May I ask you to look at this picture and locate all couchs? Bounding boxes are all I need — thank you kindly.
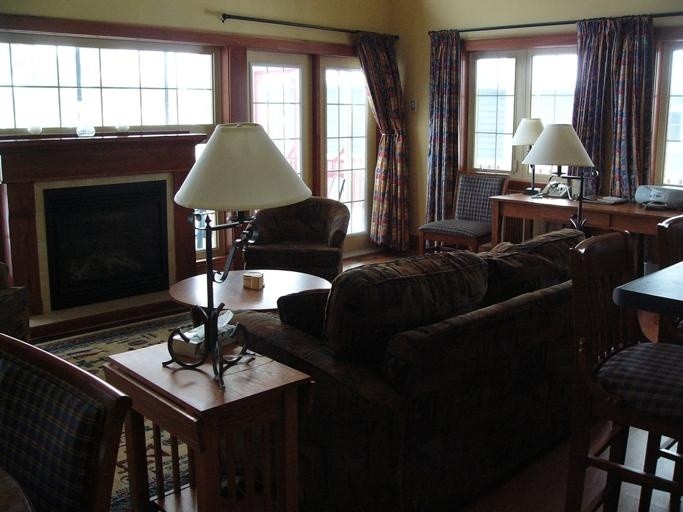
[227,227,585,512]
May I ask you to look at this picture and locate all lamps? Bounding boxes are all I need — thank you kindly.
[163,124,312,390]
[510,118,545,195]
[519,124,600,231]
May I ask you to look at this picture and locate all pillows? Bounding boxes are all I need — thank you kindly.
[323,249,489,346]
[475,227,585,306]
[277,289,330,331]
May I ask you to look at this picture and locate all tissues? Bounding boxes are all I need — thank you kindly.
[169,310,236,359]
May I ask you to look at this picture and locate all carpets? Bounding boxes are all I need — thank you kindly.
[30,310,195,511]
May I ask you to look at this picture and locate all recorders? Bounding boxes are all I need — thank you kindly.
[635,185,683,210]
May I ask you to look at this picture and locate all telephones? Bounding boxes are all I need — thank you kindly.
[542,181,570,198]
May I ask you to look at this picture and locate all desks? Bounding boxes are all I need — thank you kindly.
[489,192,683,250]
[611,260,683,512]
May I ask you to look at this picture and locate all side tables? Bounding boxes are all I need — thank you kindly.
[103,340,313,512]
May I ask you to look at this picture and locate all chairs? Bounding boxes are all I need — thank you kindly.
[0,332,132,512]
[418,171,510,258]
[638,215,683,268]
[565,230,683,512]
[241,196,350,282]
